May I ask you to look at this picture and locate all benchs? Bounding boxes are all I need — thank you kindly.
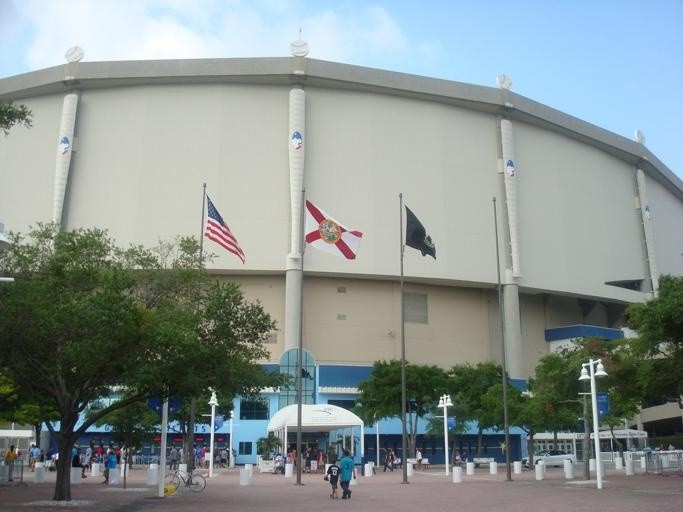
[406,458,431,471]
[472,456,494,466]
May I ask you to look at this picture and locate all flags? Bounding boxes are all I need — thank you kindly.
[204,194,245,263]
[304,199,363,262]
[404,203,436,260]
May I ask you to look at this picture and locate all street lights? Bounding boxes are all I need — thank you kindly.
[577,358,609,490]
[437,393,455,476]
[206,386,220,479]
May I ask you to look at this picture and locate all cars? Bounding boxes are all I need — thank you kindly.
[521,448,576,470]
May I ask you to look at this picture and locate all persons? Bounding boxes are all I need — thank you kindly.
[73,440,123,483]
[416,446,423,471]
[324,459,341,499]
[272,446,325,474]
[170,443,228,470]
[383,447,397,472]
[28,444,43,471]
[339,450,357,499]
[5,446,18,481]
[45,447,53,472]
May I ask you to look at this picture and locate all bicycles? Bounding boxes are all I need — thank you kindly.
[163,460,206,496]
